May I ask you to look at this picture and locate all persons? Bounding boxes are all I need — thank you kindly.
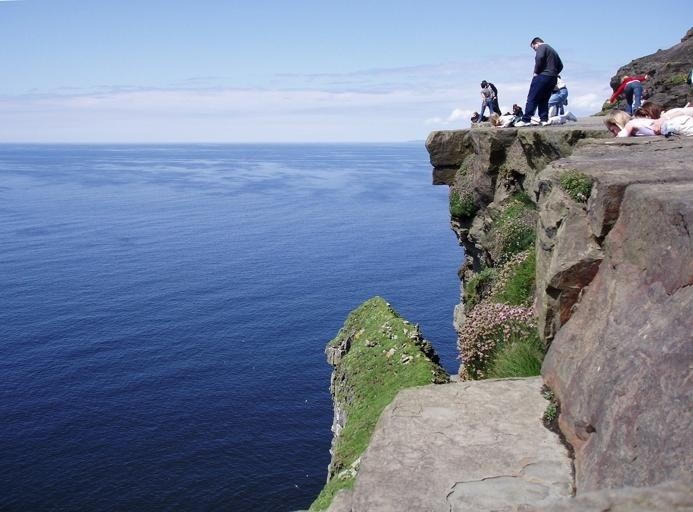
[602,75,693,138]
[471,75,577,128]
[514,37,563,127]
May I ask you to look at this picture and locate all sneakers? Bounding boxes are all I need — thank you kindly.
[567,111,577,122]
[514,121,531,128]
